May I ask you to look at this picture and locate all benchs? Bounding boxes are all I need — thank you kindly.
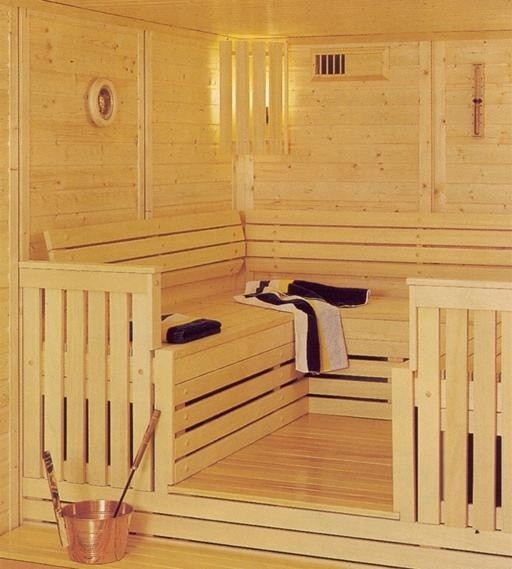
[242,210,512,419]
[44,210,309,483]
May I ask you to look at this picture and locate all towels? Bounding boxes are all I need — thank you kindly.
[130,313,222,344]
[233,293,349,375]
[245,280,369,308]
